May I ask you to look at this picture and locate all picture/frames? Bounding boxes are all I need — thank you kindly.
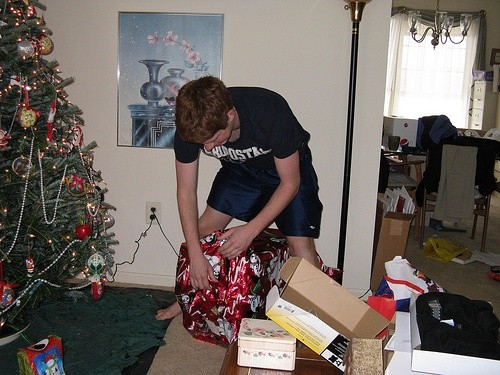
[117,11,224,149]
[490,48,500,65]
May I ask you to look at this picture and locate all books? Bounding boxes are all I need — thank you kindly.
[382,184,415,215]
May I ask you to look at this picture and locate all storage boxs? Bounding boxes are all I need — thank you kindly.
[382,115,418,150]
[371,199,416,291]
[473,70,493,81]
[238,257,387,375]
[384,297,500,375]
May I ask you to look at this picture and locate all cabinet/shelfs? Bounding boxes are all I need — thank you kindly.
[471,80,498,130]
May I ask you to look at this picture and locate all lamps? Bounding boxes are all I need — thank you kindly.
[410,0,473,50]
[337,0,373,286]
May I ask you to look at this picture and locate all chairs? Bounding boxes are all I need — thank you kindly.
[422,136,500,252]
[380,149,425,243]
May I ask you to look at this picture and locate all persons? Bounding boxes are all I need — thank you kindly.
[156,76,323,323]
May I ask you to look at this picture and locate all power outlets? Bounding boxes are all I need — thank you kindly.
[146,202,162,224]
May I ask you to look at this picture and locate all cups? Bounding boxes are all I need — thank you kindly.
[388,136,400,150]
[383,135,389,149]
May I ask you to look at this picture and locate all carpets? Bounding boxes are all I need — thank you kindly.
[0,283,177,375]
